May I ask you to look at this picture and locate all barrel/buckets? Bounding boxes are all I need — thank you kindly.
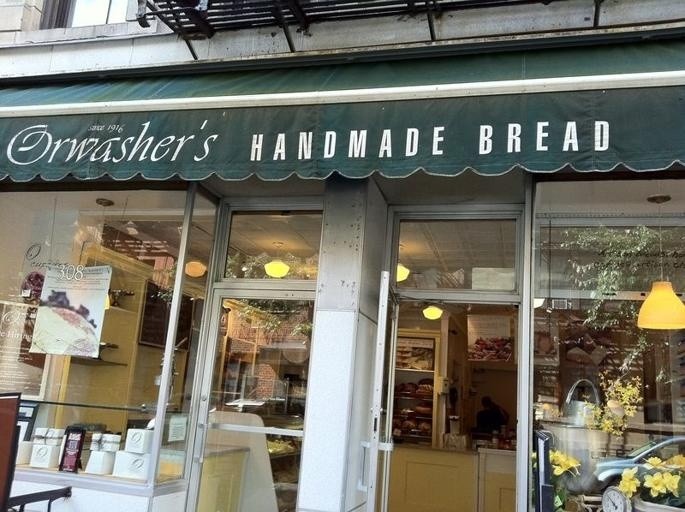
[540,424,609,495]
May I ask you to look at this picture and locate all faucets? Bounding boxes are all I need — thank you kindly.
[565,376,601,408]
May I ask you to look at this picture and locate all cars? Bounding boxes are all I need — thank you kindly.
[209,398,304,440]
[591,433,685,496]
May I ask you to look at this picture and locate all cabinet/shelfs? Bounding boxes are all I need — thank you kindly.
[48,252,279,452]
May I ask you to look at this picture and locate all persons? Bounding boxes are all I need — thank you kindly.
[477,395,506,437]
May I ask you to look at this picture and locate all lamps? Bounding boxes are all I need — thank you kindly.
[637,194,685,330]
